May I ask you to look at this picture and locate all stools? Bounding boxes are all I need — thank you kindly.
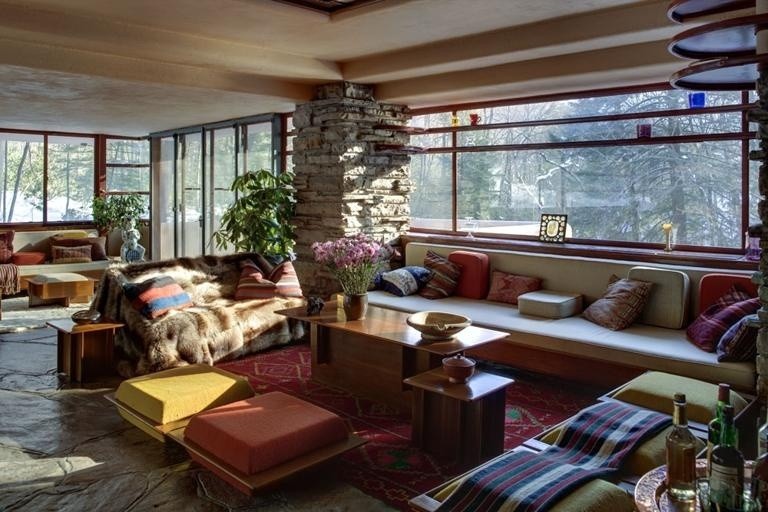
[24,273,95,308]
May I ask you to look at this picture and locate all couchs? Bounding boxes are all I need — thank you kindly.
[365,238,766,395]
[0,229,114,296]
[90,254,308,381]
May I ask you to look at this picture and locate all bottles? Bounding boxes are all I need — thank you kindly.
[749,453,768,511]
[706,383,740,484]
[758,423,768,457]
[666,393,697,502]
[710,406,744,512]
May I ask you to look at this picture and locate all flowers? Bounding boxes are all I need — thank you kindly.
[311,236,400,295]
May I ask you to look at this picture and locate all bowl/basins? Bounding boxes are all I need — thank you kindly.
[442,354,476,383]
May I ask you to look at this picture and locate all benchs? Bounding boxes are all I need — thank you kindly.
[103,364,371,499]
[403,370,749,512]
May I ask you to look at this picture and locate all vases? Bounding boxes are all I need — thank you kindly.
[343,293,370,324]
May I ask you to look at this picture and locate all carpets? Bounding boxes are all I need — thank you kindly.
[0,294,100,334]
[209,340,597,512]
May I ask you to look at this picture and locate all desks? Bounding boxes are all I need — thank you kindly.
[45,311,125,385]
[402,363,515,467]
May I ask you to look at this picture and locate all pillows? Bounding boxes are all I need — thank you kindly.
[0,229,110,264]
[123,274,193,319]
[233,255,305,299]
